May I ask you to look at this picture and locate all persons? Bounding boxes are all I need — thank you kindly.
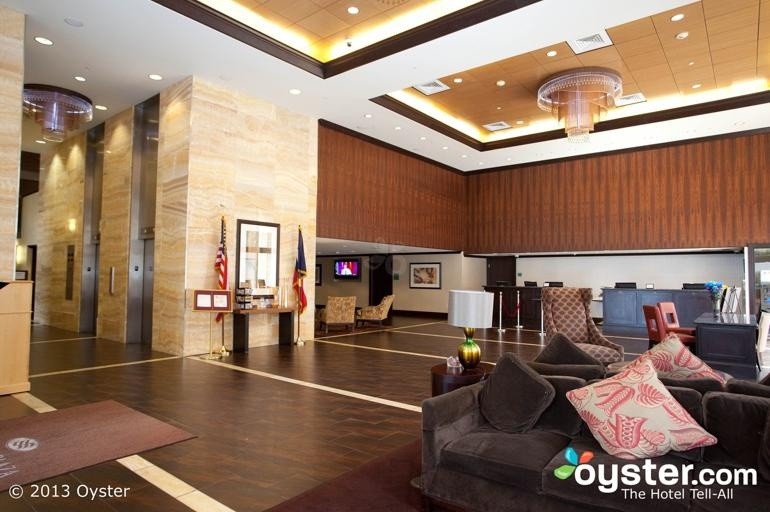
[340,261,353,276]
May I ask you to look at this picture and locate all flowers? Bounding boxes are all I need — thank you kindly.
[704,280,724,312]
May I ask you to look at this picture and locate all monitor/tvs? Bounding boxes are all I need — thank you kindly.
[334,258,360,280]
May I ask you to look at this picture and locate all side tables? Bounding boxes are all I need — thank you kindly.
[431,364,496,397]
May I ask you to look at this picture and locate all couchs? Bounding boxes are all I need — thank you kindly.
[420,360,770,512]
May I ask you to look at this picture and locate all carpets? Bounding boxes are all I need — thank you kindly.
[264,438,482,512]
[0,398,198,493]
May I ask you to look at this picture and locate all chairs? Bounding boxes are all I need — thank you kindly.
[524,281,537,287]
[545,282,563,287]
[541,287,624,366]
[496,281,510,287]
[614,283,636,289]
[682,283,706,290]
[643,302,697,357]
[356,295,397,329]
[317,296,357,334]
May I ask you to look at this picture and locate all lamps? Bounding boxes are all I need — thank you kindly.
[448,290,495,370]
[23,82,94,143]
[536,66,623,145]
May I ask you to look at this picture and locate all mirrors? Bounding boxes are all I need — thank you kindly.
[236,219,280,300]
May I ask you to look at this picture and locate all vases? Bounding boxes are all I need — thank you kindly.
[711,299,721,322]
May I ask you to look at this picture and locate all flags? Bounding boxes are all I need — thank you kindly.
[214,219,230,324]
[291,230,308,313]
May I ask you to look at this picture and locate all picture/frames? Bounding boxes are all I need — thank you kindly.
[409,262,441,289]
[315,263,322,287]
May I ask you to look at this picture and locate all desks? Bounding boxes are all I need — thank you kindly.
[693,312,759,381]
[232,308,297,353]
